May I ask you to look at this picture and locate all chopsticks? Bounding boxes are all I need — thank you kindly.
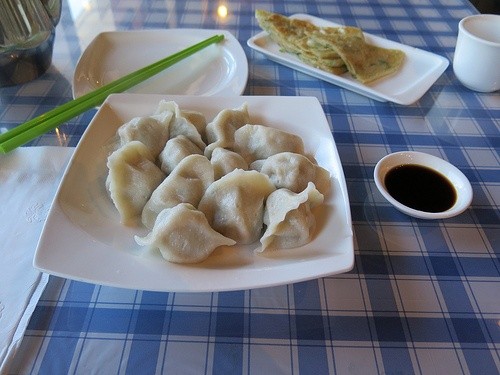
[0,34,227,153]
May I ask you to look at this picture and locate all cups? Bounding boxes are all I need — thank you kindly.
[452,15,500,92]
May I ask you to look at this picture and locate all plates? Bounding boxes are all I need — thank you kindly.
[72,28,249,112]
[35,94,354,292]
[372,151,475,218]
[246,13,449,105]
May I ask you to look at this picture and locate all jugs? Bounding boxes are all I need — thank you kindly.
[0,1,62,86]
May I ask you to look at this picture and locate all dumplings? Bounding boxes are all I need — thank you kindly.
[105,97,328,263]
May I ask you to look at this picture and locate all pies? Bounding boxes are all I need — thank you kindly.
[256,8,407,84]
